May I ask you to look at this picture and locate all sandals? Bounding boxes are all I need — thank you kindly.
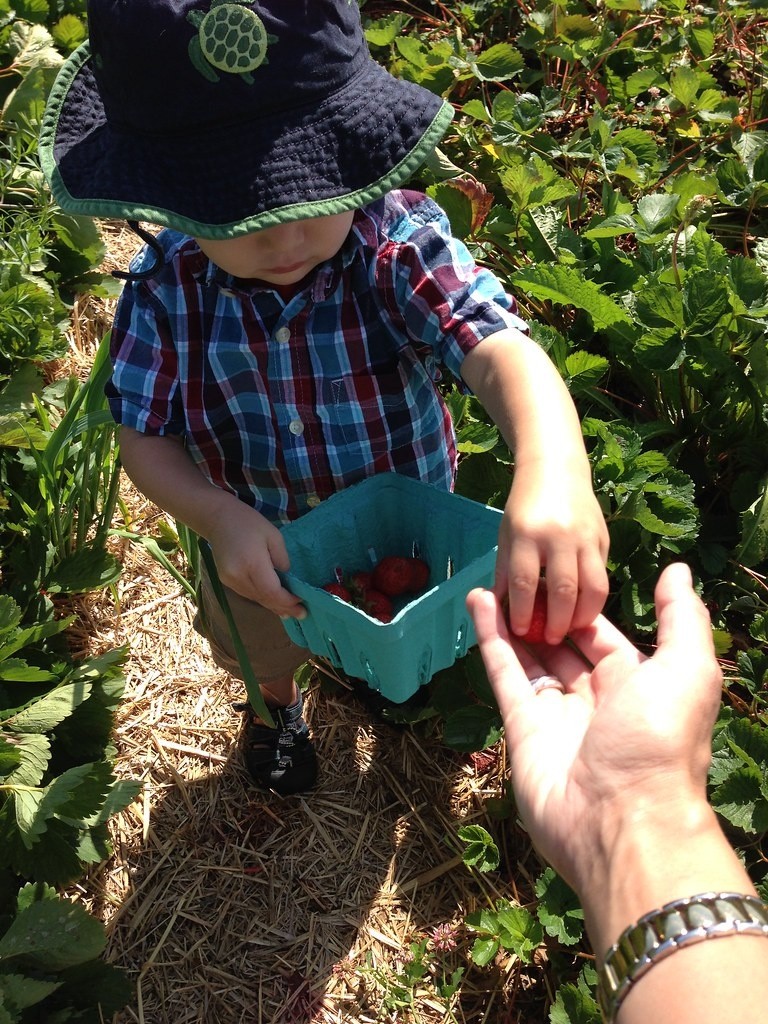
[347,678,432,727]
[232,683,317,795]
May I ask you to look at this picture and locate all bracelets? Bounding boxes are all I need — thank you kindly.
[595,890,768,1024]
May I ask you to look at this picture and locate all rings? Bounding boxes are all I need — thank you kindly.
[530,673,566,694]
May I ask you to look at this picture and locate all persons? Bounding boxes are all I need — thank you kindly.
[39,1,610,796]
[465,561,767,1024]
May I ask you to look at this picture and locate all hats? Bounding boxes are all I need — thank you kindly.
[39,0,456,241]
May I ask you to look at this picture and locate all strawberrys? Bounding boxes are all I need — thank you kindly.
[506,579,548,644]
[320,555,429,624]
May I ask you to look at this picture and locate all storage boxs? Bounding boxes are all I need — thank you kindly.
[273,473,507,703]
